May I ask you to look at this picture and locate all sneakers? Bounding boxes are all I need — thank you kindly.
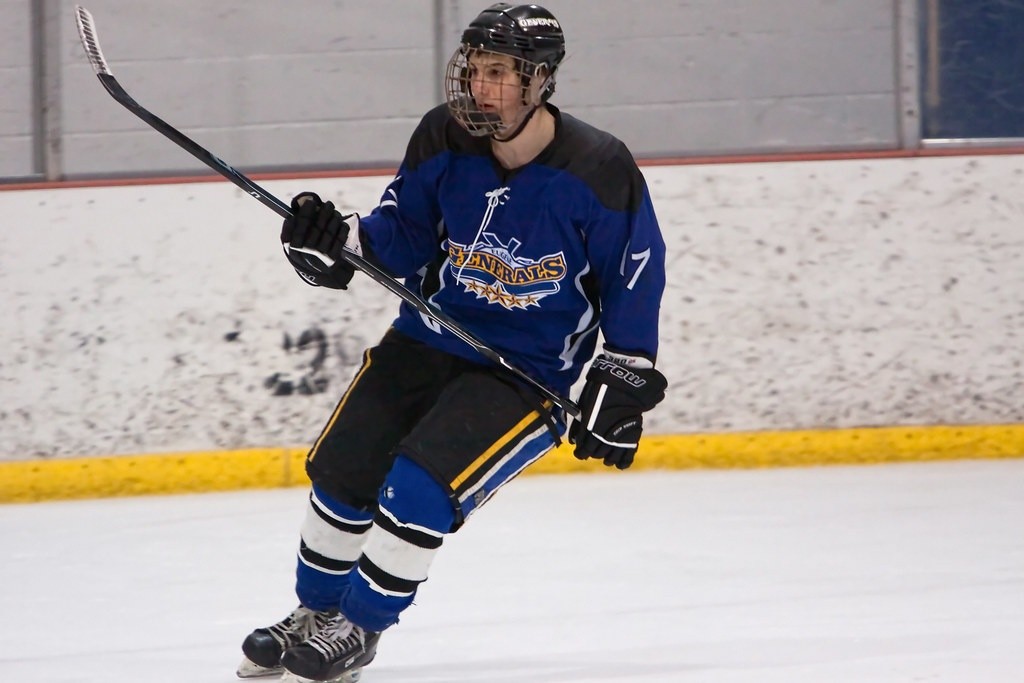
[236,606,338,677]
[279,612,381,683]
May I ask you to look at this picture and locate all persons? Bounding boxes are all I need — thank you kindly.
[236,0,667,683]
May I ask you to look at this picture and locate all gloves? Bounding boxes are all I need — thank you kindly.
[280,192,357,289]
[568,354,667,470]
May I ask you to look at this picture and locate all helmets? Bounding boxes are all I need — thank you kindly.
[445,3,566,137]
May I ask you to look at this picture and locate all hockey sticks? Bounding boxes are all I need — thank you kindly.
[71,3,584,422]
